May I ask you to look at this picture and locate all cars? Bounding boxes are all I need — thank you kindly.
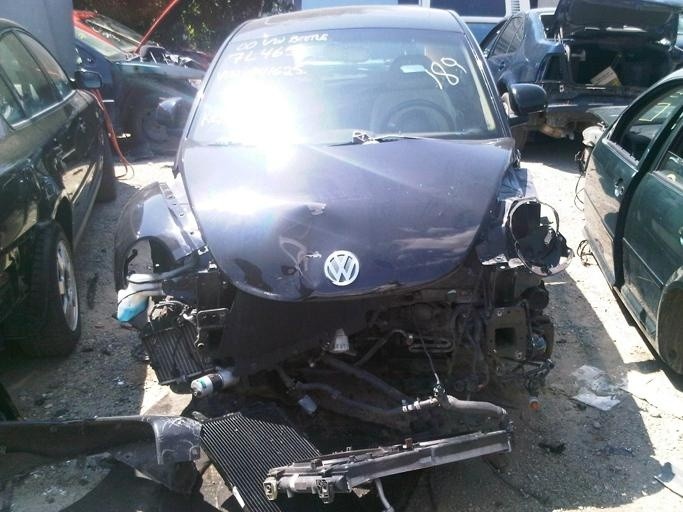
[574,67,683,378]
[479,0,682,156]
[73,0,214,156]
[460,16,505,44]
[113,5,581,430]
[1,18,117,360]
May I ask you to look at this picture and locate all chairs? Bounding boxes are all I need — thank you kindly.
[368,55,457,134]
